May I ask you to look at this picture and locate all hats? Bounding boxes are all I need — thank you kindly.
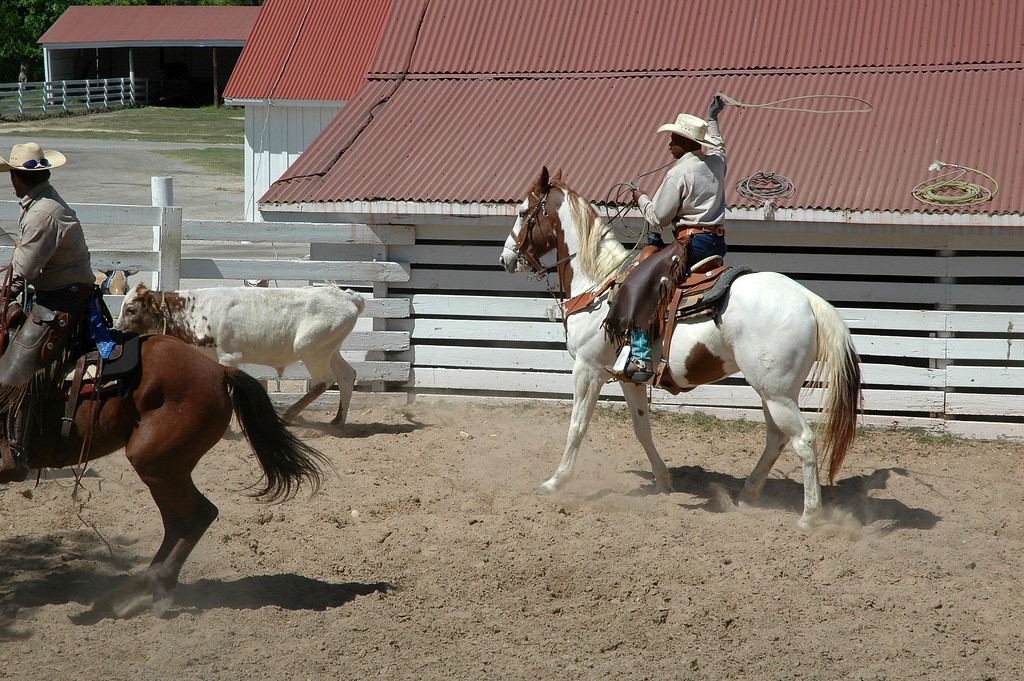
[655,111,717,150]
[0,143,68,173]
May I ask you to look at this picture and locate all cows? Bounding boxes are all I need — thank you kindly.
[113,282,366,439]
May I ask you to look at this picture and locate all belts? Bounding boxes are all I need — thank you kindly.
[671,225,727,240]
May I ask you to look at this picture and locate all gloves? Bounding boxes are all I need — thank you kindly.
[707,95,724,120]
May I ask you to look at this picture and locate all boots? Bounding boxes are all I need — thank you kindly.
[603,357,655,383]
[1,403,31,482]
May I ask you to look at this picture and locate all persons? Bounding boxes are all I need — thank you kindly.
[604,94,729,384]
[1,138,98,485]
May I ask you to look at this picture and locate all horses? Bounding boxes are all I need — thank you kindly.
[497,166,862,529]
[0,295,344,623]
